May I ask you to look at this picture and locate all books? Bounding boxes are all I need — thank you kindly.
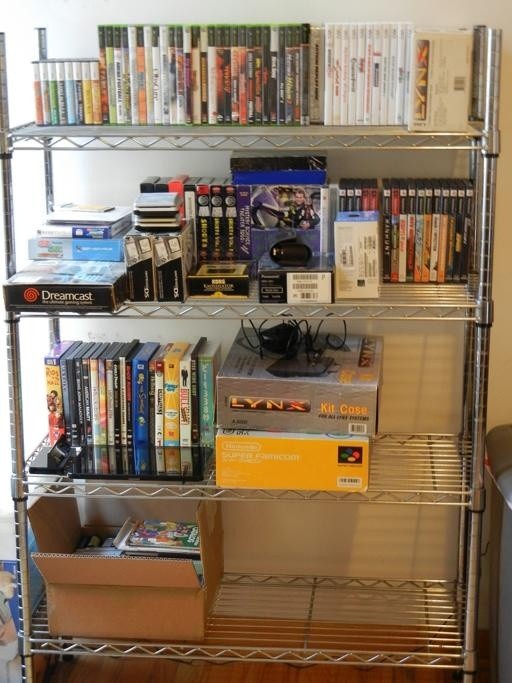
[214,324,385,434]
[230,156,327,280]
[216,429,368,492]
[44,342,220,480]
[29,205,132,262]
[321,178,474,283]
[217,328,383,435]
[215,430,371,493]
[25,474,223,644]
[74,522,202,576]
[31,24,474,132]
[126,175,235,301]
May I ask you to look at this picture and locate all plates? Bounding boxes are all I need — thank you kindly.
[0,23,503,683]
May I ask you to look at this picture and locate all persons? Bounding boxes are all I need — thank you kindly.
[278,191,320,229]
